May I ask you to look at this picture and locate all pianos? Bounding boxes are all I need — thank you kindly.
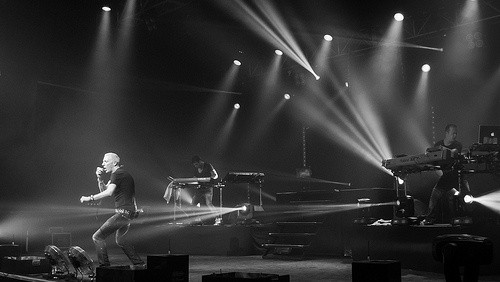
[223,171,265,184]
[382,143,458,173]
[167,176,213,189]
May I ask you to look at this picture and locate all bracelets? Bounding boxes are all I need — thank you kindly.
[91,195,94,201]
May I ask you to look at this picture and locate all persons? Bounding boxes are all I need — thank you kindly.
[420,125,470,225]
[191,156,222,226]
[80,152,146,278]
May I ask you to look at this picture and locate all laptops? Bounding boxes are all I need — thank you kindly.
[478,125,500,145]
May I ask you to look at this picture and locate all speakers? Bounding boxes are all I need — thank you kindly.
[352,260,402,282]
[95,254,189,282]
